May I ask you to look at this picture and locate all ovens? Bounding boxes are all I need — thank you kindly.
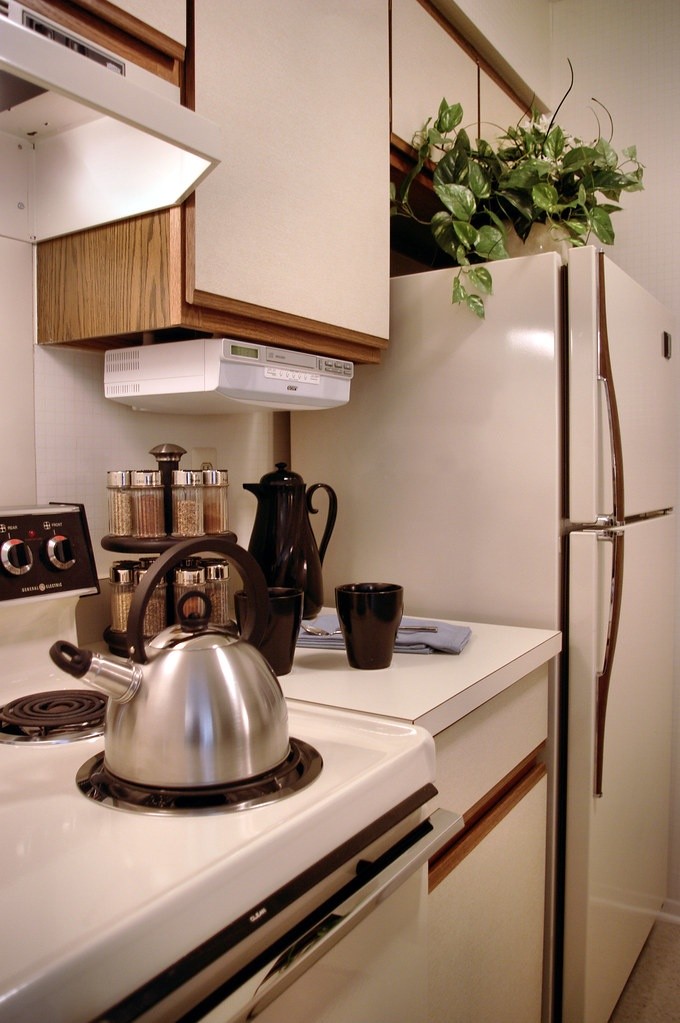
[128,784,464,1023]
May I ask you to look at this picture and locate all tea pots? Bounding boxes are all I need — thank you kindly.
[243,462,337,621]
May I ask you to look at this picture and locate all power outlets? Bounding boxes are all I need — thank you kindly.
[190,447,217,471]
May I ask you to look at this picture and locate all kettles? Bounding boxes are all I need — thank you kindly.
[49,537,291,788]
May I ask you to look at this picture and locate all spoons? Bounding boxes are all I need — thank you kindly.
[301,622,438,636]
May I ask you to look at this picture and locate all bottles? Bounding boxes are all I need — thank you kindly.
[171,470,230,537]
[174,556,230,627]
[108,470,164,537]
[110,558,165,637]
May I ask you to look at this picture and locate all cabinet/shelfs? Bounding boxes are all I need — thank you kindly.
[429,659,556,1023]
[0,0,397,364]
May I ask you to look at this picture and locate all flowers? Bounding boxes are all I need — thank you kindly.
[393,55,647,319]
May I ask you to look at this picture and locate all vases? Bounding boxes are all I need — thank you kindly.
[501,216,573,264]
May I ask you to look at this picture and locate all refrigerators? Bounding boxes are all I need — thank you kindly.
[285,244,680,1023]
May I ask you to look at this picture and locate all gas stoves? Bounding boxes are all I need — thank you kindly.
[0,502,438,1023]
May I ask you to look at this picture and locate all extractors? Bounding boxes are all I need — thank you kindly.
[0,0,226,244]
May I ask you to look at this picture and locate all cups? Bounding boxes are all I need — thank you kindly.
[233,588,304,676]
[335,582,403,671]
